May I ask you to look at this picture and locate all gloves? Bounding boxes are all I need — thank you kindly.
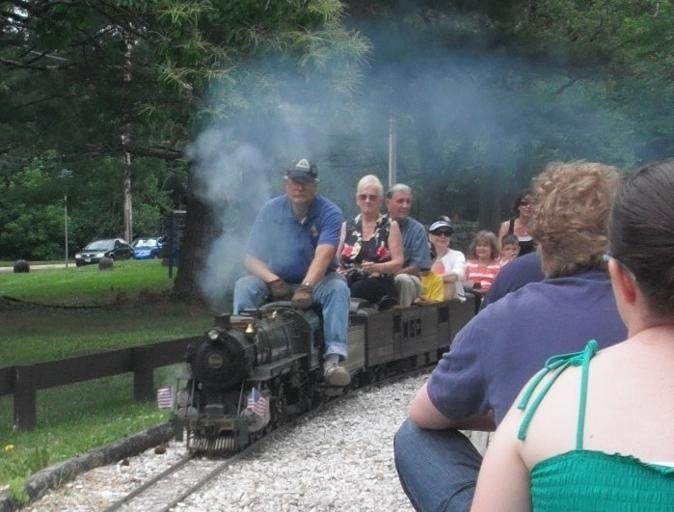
[291,284,314,311]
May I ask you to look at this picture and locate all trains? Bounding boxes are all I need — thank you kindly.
[169,286,484,460]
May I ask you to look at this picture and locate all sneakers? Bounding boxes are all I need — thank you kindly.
[323,364,351,398]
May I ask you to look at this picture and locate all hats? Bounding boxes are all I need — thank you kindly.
[427,215,454,232]
[285,156,318,183]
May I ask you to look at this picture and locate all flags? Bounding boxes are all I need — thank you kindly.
[247,388,265,416]
[157,388,171,410]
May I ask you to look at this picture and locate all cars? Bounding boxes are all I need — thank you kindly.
[74,235,168,267]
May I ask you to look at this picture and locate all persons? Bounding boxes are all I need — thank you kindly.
[469,158,674,512]
[233,158,351,387]
[394,160,630,512]
[336,175,544,312]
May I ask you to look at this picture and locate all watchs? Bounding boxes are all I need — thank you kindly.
[301,281,313,289]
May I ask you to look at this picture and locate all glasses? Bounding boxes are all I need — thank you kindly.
[431,229,453,236]
[519,201,533,206]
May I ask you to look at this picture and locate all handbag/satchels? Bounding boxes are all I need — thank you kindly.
[267,278,292,301]
